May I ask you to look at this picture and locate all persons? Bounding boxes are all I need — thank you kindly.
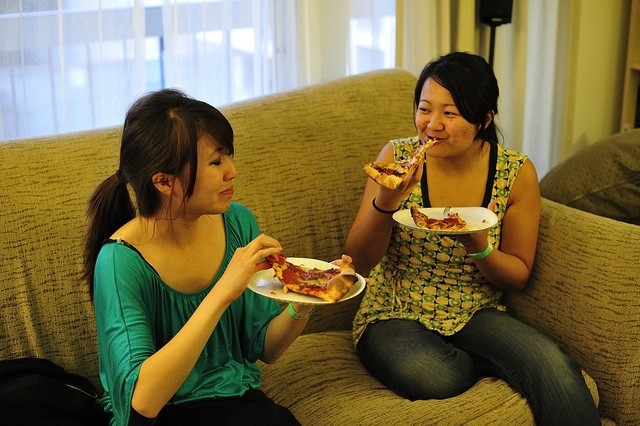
[80,89,354,426]
[345,51,601,426]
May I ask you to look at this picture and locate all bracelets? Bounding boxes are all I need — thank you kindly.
[286,302,317,321]
[372,197,402,215]
[467,244,493,260]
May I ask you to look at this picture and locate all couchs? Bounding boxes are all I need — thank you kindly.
[0,71,640,425]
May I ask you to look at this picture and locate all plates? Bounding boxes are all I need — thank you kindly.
[247,257,367,306]
[392,206,499,235]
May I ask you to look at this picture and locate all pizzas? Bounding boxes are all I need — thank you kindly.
[364,138,440,189]
[263,251,355,304]
[410,204,467,232]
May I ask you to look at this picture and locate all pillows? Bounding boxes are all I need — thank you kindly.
[540,126,640,226]
